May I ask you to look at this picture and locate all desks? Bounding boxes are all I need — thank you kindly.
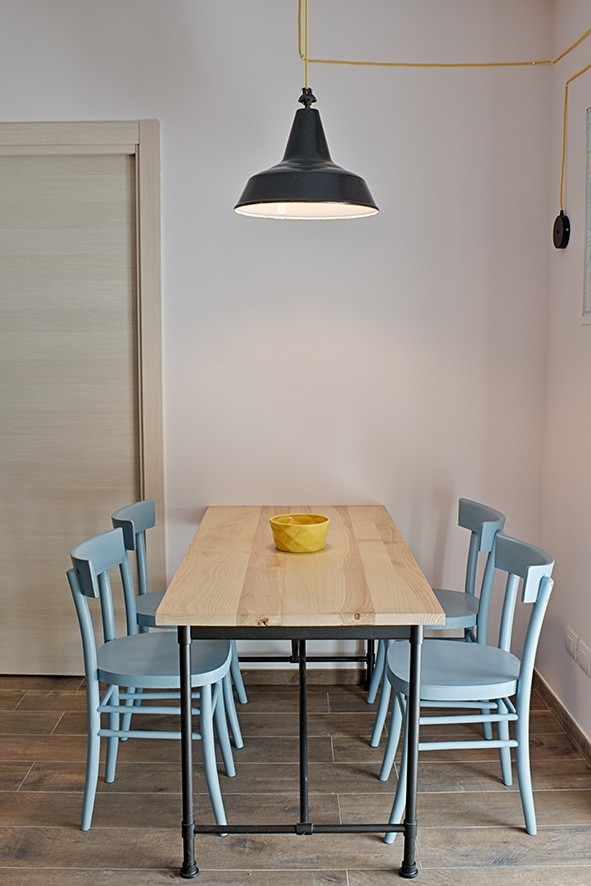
[155,504,446,881]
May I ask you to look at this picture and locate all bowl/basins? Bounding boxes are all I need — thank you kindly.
[269,513,330,553]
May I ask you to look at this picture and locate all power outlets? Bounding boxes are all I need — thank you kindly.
[564,626,591,677]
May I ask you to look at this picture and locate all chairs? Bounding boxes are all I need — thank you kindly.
[380,534,555,844]
[112,498,247,750]
[367,499,506,746]
[66,527,230,838]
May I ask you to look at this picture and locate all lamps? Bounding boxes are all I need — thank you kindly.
[233,0,380,220]
